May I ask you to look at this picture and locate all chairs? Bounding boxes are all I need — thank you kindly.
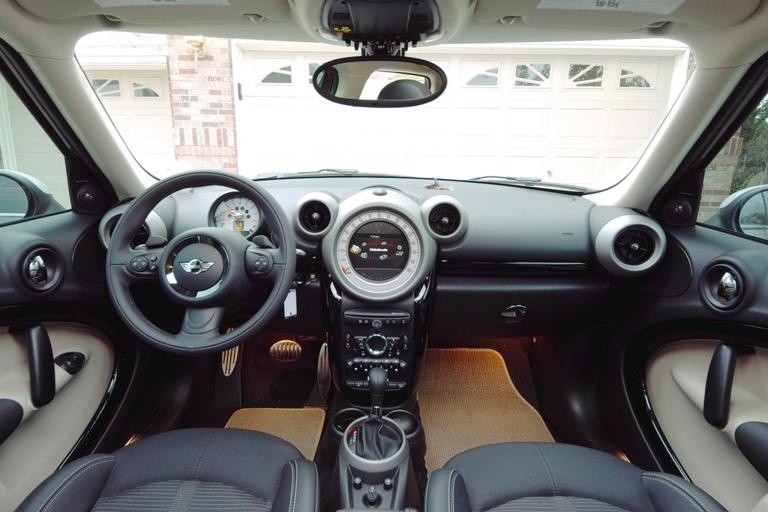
[422,440,729,511]
[14,427,321,512]
